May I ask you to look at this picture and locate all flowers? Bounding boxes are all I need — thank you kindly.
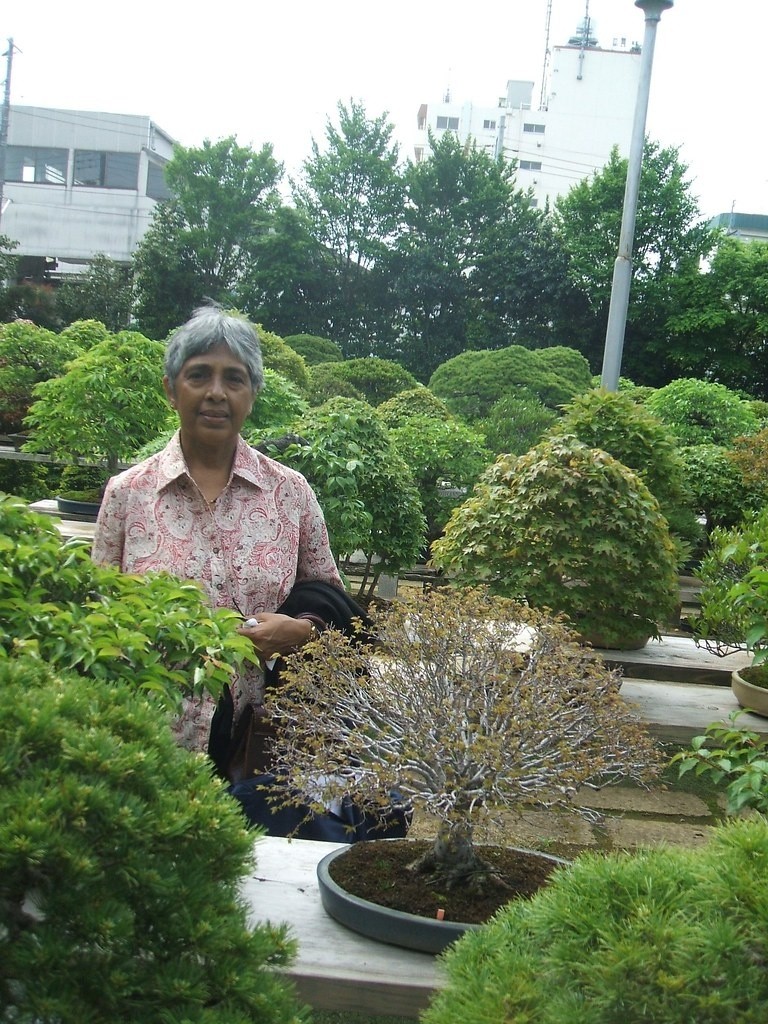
[253,585,671,898]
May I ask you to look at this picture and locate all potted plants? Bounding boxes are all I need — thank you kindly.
[21,342,172,515]
[688,504,768,716]
[426,435,679,649]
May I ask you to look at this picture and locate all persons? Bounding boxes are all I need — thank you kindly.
[91,307,343,794]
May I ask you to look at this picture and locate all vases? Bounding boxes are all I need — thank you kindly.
[318,838,574,952]
